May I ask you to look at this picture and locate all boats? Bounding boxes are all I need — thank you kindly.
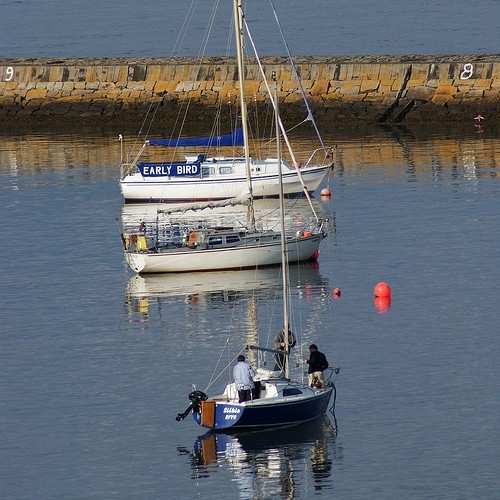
[177,418,336,500]
[169,68,345,434]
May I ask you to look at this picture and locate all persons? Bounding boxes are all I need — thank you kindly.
[304,344,329,389]
[274,323,296,372]
[232,355,255,404]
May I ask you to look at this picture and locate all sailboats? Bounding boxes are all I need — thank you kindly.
[125,261,329,369]
[122,198,329,237]
[119,1,338,201]
[116,1,332,277]
